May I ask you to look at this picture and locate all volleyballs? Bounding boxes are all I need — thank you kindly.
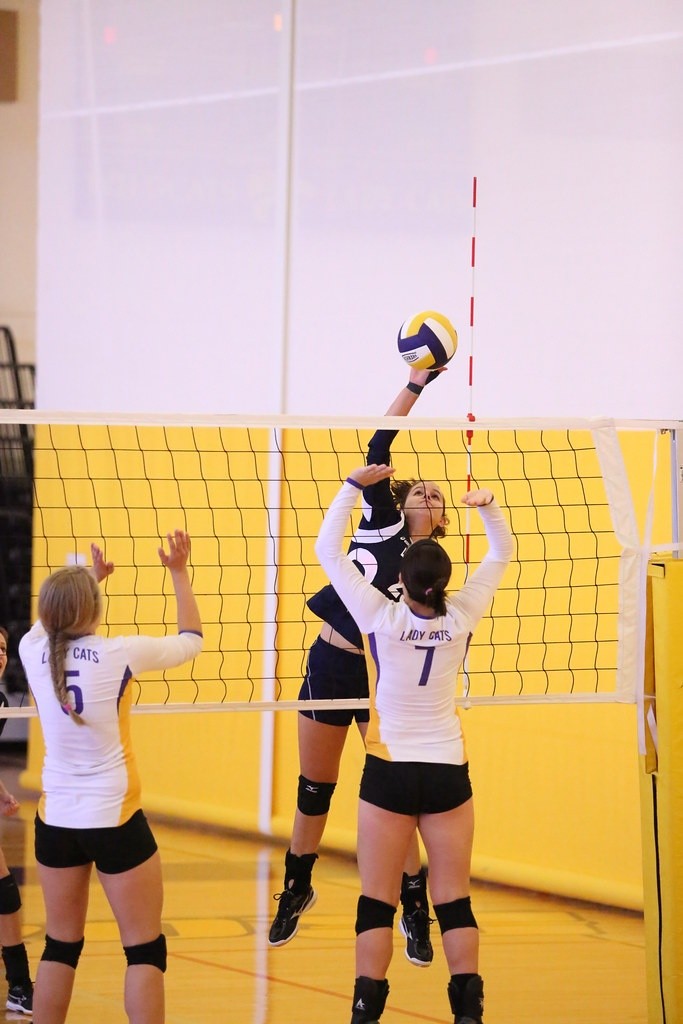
[396,309,459,372]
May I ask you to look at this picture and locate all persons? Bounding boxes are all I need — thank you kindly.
[267,363,451,967]
[0,627,36,1016]
[18,529,205,1024]
[314,463,512,1024]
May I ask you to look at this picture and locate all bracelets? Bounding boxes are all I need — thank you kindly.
[405,381,424,395]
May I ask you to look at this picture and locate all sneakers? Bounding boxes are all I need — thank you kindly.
[268,883,319,947]
[398,900,434,967]
[5,987,33,1014]
